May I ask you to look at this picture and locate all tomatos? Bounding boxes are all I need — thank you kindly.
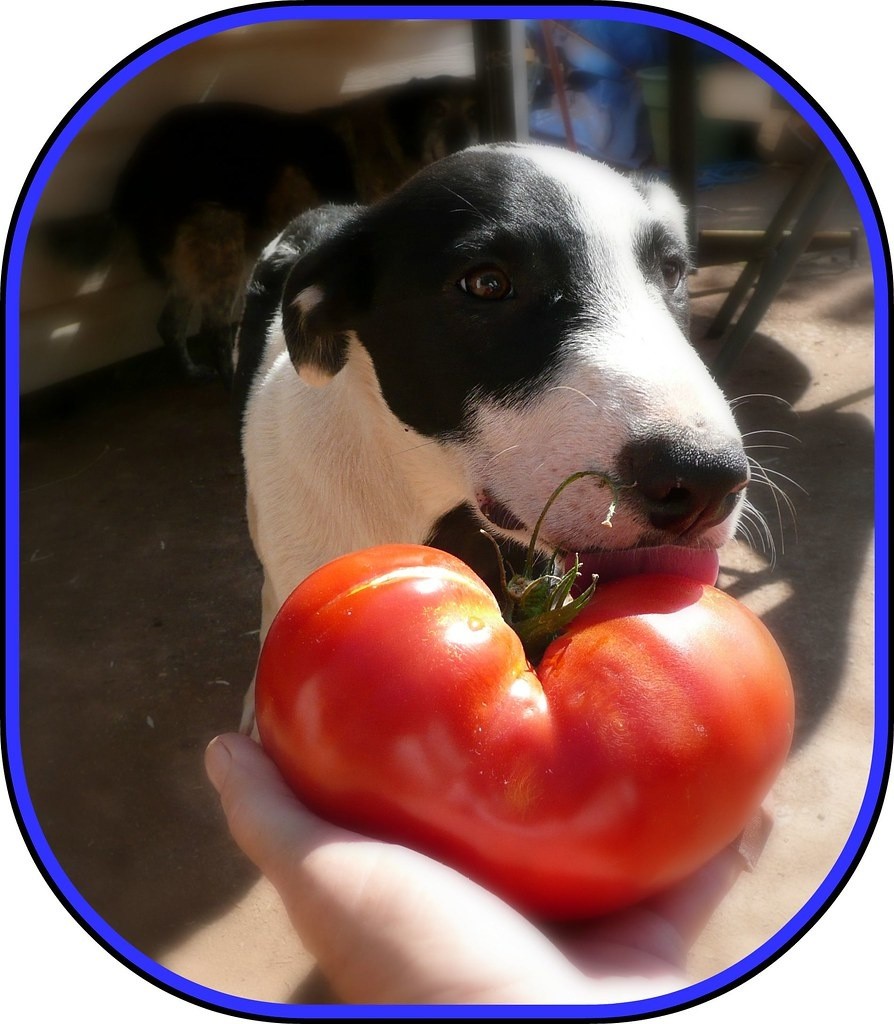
[253,465,793,927]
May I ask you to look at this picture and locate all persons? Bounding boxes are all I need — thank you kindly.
[205,735,773,1004]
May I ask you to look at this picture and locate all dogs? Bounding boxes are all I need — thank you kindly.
[30,68,749,760]
